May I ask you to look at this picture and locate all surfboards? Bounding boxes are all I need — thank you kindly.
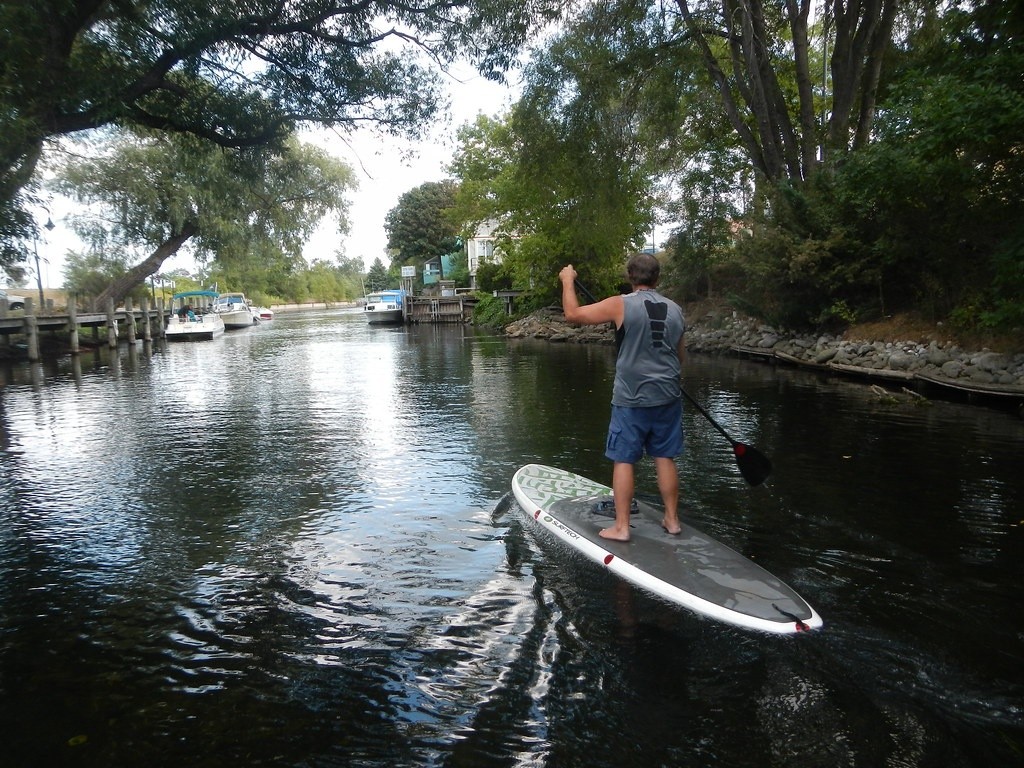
[510,463,824,635]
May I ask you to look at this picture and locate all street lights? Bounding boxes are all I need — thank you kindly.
[27,205,56,312]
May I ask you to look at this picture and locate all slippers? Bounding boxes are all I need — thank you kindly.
[630,498,639,514]
[591,499,616,517]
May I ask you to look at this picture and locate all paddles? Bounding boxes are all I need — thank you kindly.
[572,278,774,488]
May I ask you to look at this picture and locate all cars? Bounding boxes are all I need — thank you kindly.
[0,290,35,313]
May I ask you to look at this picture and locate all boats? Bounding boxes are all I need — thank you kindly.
[362,277,407,326]
[212,281,256,328]
[162,290,228,342]
[250,306,280,322]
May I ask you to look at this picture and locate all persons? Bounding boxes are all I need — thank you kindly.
[558,252,683,540]
[178,303,213,322]
